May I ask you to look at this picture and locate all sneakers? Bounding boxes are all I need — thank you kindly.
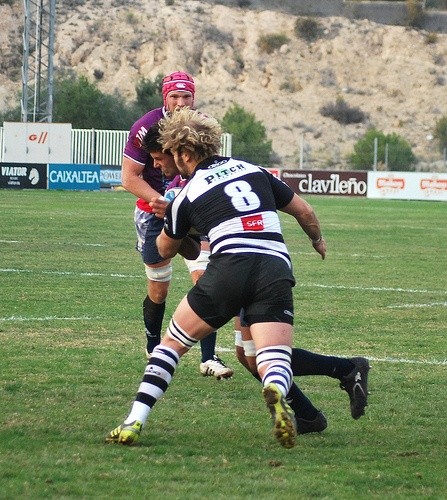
[339,357,372,420]
[262,383,297,449]
[146,348,153,364]
[199,355,233,380]
[293,409,327,434]
[105,420,143,444]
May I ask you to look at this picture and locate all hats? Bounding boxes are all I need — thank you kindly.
[162,72,194,110]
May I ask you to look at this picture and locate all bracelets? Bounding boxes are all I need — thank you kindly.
[309,235,323,243]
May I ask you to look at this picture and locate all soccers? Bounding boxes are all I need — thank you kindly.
[163,187,183,203]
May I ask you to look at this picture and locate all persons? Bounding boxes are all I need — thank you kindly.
[102,104,328,447]
[142,127,372,434]
[121,71,236,383]
[377,160,392,171]
